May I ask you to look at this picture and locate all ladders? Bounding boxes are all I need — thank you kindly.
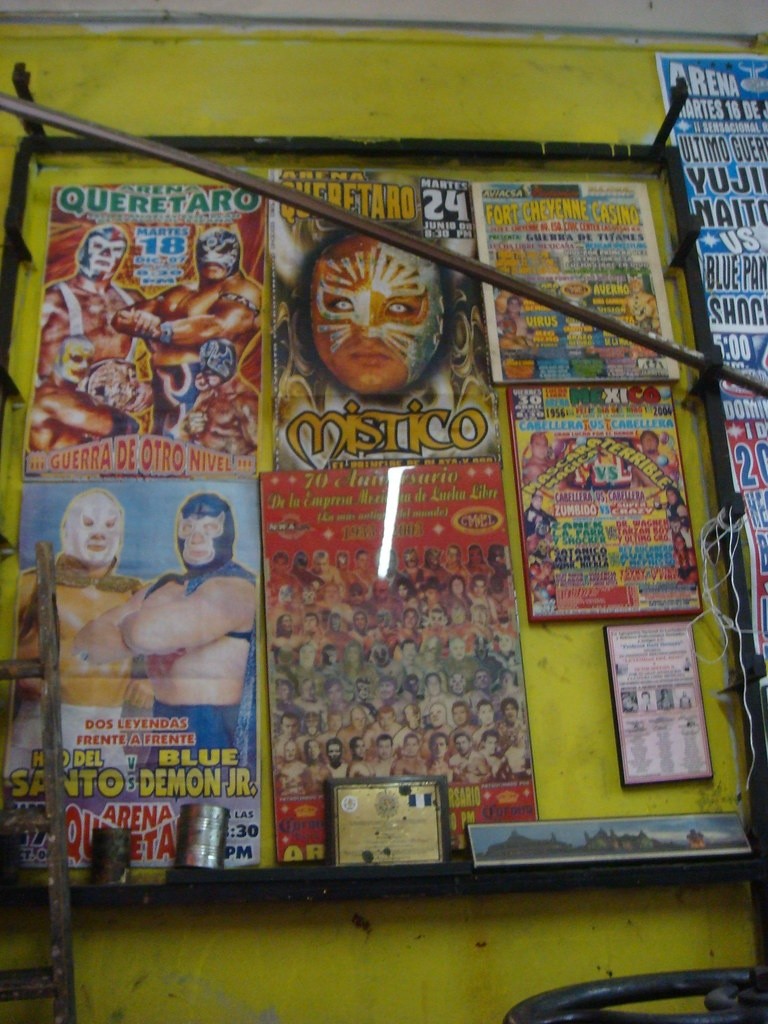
[1,540,80,1024]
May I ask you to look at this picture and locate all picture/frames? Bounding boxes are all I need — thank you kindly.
[464,810,754,873]
[323,774,452,866]
[603,622,715,790]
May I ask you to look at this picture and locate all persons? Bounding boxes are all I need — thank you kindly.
[14,226,531,792]
[634,431,697,582]
[622,688,692,712]
[523,432,557,600]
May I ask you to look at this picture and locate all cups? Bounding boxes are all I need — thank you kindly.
[175,804,229,871]
